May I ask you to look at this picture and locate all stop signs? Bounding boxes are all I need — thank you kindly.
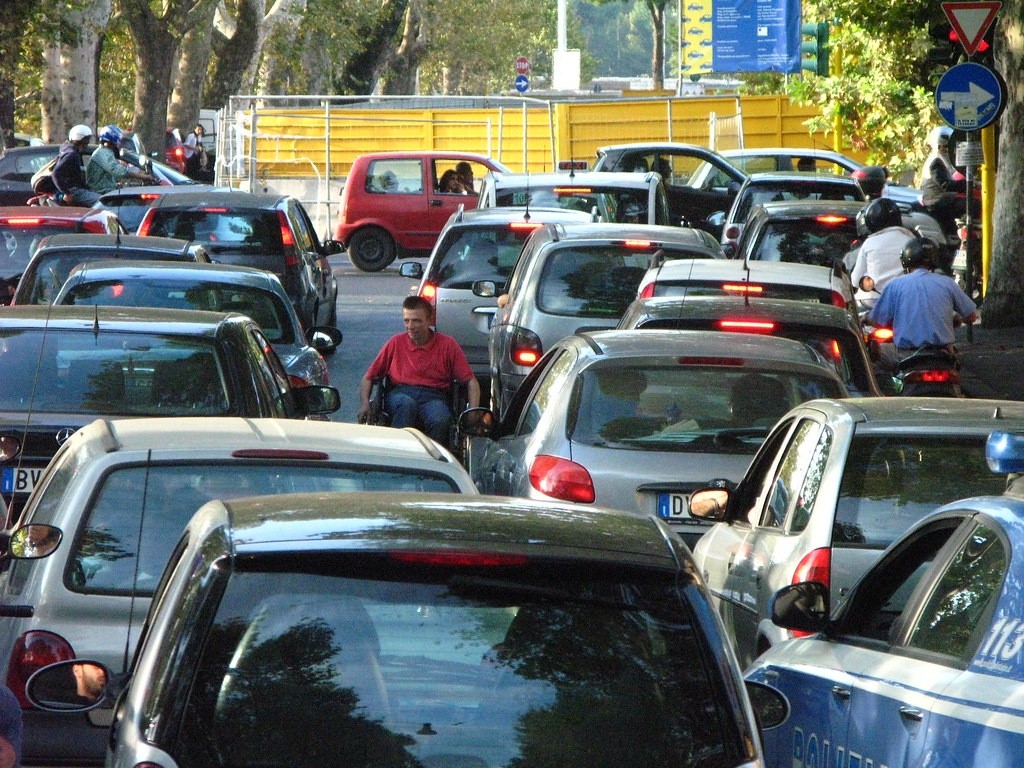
[517,56,530,75]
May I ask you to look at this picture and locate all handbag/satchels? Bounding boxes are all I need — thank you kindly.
[31,153,60,196]
[200,150,207,166]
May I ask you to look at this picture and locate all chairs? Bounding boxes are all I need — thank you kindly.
[217,603,389,768]
[68,359,124,402]
[0,344,59,402]
[862,448,909,529]
[175,213,209,241]
[777,233,815,262]
[591,372,647,430]
[91,477,189,585]
[149,353,216,409]
[481,600,669,768]
[728,374,791,430]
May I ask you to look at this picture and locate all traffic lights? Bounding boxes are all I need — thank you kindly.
[799,21,830,79]
[975,19,1000,64]
[930,20,959,67]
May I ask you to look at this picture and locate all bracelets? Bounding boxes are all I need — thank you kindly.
[711,497,719,516]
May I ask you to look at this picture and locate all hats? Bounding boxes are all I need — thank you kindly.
[99,125,125,146]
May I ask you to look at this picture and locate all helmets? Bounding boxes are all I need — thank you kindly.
[865,198,902,233]
[928,126,954,154]
[69,125,92,142]
[852,165,886,199]
[901,237,939,269]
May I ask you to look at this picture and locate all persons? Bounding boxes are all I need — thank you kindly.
[27,525,61,558]
[858,238,976,360]
[73,664,106,706]
[52,125,100,208]
[850,199,917,318]
[693,496,728,518]
[86,125,154,194]
[860,276,873,291]
[464,239,498,275]
[798,158,816,172]
[920,126,967,277]
[359,296,480,447]
[651,158,711,190]
[440,162,477,194]
[380,171,410,193]
[0,437,19,462]
[184,124,203,180]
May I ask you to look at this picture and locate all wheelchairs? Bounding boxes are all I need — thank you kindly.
[361,374,467,470]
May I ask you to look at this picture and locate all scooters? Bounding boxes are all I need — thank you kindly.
[857,271,980,398]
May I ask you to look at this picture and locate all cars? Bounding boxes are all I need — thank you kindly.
[747,422,1024,767]
[686,396,1024,681]
[24,488,792,768]
[1,95,982,559]
[0,415,513,768]
